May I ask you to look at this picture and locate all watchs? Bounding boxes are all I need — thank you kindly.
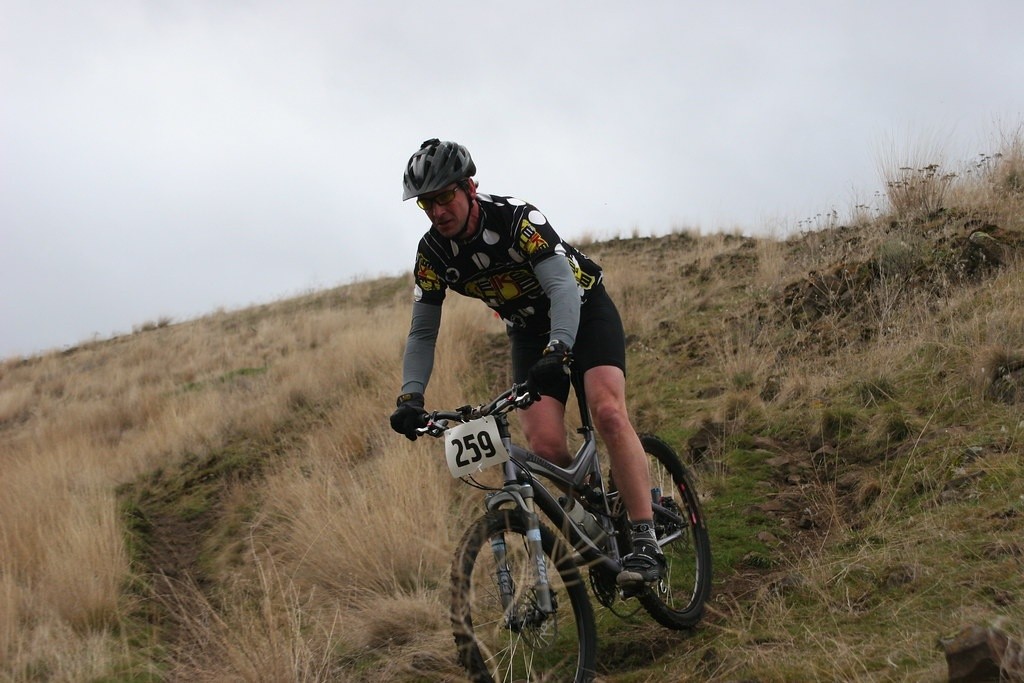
[547,340,563,347]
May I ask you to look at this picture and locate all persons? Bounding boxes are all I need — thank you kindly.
[389,138,669,583]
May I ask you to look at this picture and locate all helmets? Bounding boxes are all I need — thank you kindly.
[402,138,474,202]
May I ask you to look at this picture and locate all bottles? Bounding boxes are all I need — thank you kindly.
[557,496,614,555]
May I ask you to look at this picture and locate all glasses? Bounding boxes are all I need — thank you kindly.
[417,179,468,211]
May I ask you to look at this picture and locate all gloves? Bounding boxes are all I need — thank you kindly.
[391,393,430,441]
[528,348,566,402]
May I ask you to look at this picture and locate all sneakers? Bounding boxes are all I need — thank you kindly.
[616,536,667,586]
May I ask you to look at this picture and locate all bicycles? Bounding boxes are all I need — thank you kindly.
[413,358,712,683]
